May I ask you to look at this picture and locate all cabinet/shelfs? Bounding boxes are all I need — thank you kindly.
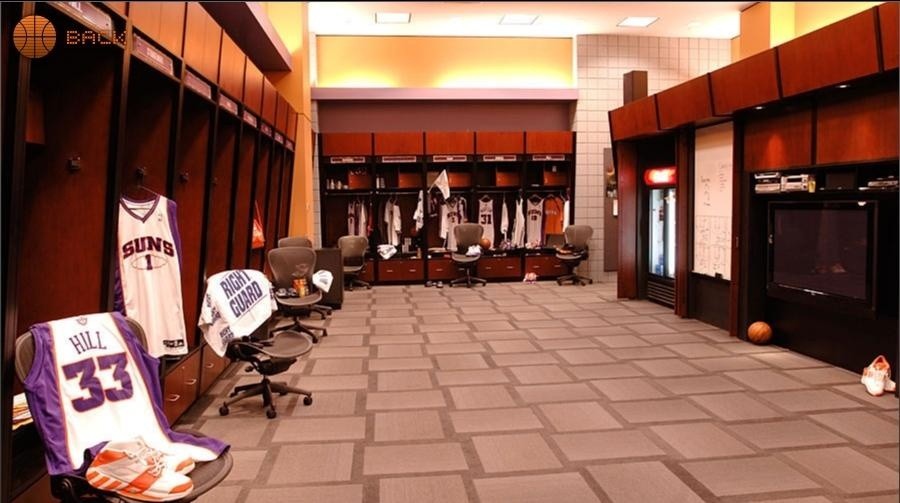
[317,129,578,283]
[1,0,298,501]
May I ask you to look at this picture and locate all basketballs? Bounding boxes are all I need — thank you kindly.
[480,239,490,248]
[13,15,56,59]
[747,322,771,344]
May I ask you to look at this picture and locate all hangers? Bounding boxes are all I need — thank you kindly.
[347,184,574,208]
[119,166,157,211]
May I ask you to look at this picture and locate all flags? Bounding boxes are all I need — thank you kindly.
[434,170,451,201]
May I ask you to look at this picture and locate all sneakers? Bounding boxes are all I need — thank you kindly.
[860,356,895,397]
[425,280,443,288]
[276,285,298,297]
[558,243,575,254]
[523,272,536,284]
[85,432,194,502]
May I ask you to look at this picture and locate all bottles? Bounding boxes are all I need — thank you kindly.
[417,248,421,258]
[809,176,816,193]
[292,276,309,298]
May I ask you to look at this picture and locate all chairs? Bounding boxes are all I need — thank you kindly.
[13,233,323,503]
[337,234,373,291]
[554,223,596,287]
[450,223,488,288]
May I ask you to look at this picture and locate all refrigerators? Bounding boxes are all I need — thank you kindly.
[643,163,676,310]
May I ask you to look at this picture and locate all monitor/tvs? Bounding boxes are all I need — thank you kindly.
[765,199,880,322]
